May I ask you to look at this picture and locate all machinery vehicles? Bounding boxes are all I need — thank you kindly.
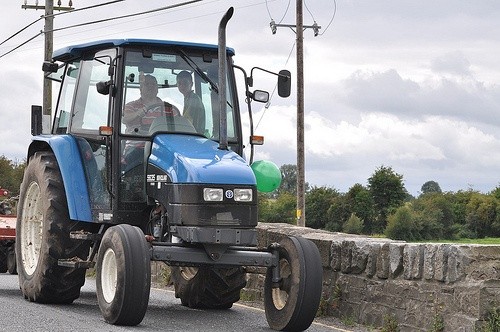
[0,185,21,274]
[13,6,324,332]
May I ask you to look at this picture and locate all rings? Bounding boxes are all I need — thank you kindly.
[138,115,140,117]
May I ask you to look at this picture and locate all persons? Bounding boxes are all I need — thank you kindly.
[176,71,205,134]
[121,74,180,171]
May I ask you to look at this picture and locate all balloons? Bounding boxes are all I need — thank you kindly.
[251,160,282,192]
